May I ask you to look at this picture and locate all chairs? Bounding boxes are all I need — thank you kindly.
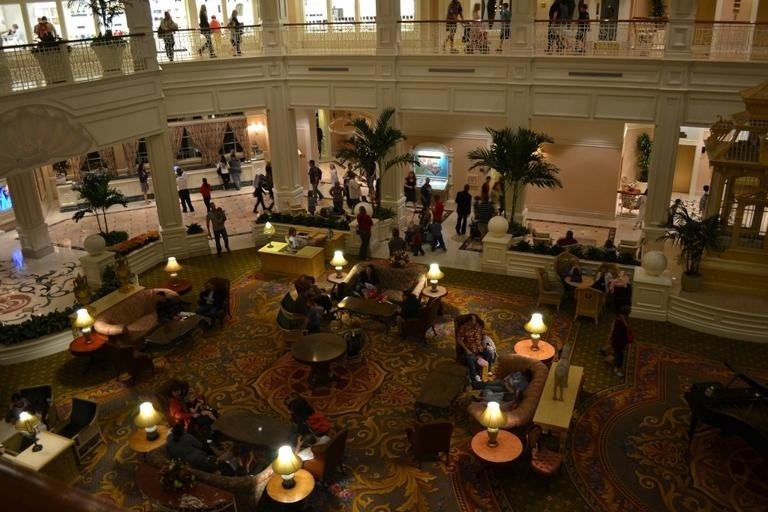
[406,420,452,465]
[398,298,441,338]
[21,383,58,432]
[534,230,640,323]
[51,397,106,465]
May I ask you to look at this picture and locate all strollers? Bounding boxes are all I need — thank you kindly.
[404,209,424,254]
[460,22,491,56]
[552,27,583,56]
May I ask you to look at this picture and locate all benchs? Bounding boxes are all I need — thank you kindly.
[156,378,211,438]
[291,423,348,484]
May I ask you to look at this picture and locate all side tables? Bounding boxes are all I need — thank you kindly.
[69,333,113,377]
[267,468,316,512]
[156,279,193,297]
[131,426,172,472]
[516,338,556,363]
[470,429,523,481]
[327,272,349,297]
[421,285,448,314]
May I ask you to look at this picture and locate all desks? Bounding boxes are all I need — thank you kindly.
[417,360,470,417]
[144,311,203,350]
[257,230,346,281]
[533,360,584,452]
[214,410,302,450]
[327,296,401,327]
[21,428,82,483]
[292,333,348,386]
[69,285,145,337]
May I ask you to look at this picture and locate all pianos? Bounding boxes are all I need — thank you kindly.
[684,361,766,449]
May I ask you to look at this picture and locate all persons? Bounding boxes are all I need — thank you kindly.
[226,10,244,56]
[556,230,577,251]
[698,184,710,219]
[632,188,648,231]
[196,5,217,59]
[176,170,195,213]
[155,291,181,324]
[276,263,421,340]
[209,15,222,50]
[195,280,226,327]
[456,314,494,381]
[7,16,58,47]
[167,384,333,474]
[316,123,324,153]
[671,198,681,218]
[591,263,633,312]
[137,163,151,205]
[597,305,632,378]
[441,0,591,55]
[160,11,177,62]
[604,239,614,248]
[5,392,33,423]
[562,255,587,310]
[474,368,534,393]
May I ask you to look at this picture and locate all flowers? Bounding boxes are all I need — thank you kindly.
[103,229,162,258]
[20,273,91,345]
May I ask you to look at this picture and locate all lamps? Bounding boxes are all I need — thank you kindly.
[425,263,444,292]
[274,445,303,490]
[330,250,346,278]
[165,256,183,285]
[135,402,163,441]
[478,401,506,447]
[75,308,96,343]
[524,314,548,350]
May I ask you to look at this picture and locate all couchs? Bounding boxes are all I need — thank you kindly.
[467,353,550,434]
[93,289,182,350]
[196,276,231,328]
[166,440,280,482]
[339,261,429,305]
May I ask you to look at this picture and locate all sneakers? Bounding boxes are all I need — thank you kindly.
[254,208,272,212]
[222,185,240,190]
[314,195,323,203]
[458,231,467,235]
[182,209,194,212]
[413,246,447,256]
[601,344,626,378]
[218,249,231,257]
